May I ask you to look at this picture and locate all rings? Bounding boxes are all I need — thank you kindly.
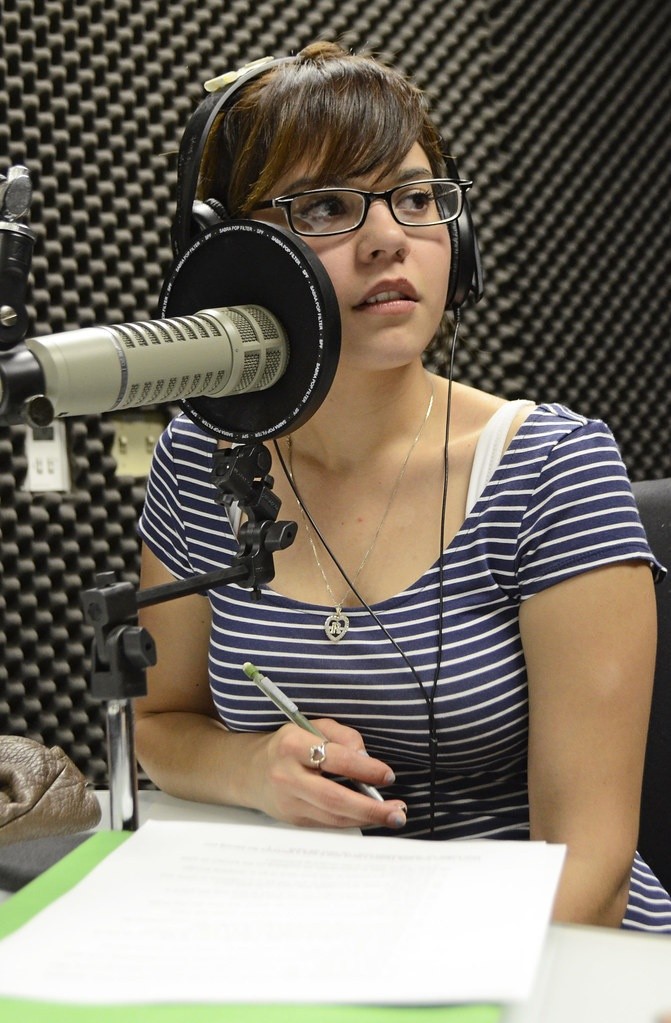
[310,740,331,772]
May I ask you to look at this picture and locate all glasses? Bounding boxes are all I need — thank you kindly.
[255,178,474,238]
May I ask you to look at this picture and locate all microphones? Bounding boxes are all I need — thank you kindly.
[0,304,289,427]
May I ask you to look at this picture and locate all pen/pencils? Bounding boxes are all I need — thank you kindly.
[242,660,383,802]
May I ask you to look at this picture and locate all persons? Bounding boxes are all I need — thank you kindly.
[130,42,671,934]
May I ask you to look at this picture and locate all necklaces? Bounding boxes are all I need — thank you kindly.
[286,375,435,643]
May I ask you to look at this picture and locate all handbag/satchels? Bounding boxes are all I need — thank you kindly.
[0,736,103,842]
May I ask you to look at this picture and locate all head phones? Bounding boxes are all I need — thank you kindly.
[170,57,483,310]
